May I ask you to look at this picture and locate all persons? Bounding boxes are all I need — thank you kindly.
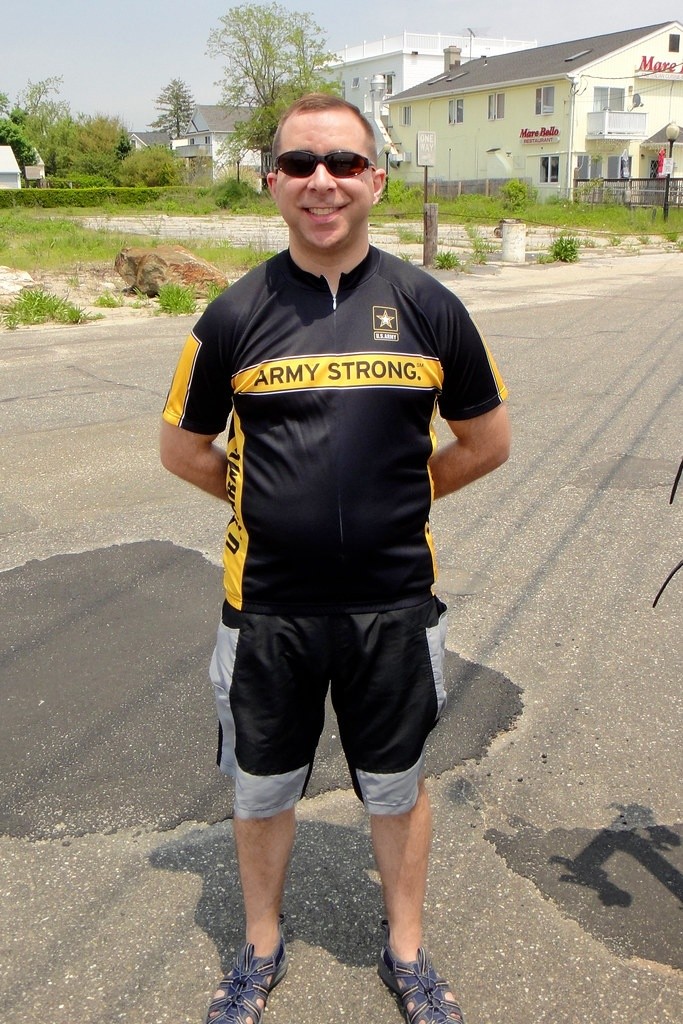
[160,94,512,1024]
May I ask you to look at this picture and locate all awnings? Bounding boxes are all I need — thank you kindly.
[641,123,683,146]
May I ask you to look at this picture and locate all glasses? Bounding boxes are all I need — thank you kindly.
[273,151,377,178]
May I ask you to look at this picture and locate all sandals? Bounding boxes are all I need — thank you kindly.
[377,919,465,1024]
[207,914,289,1024]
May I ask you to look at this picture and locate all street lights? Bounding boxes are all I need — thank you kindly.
[235,155,241,182]
[662,122,680,222]
[383,144,391,194]
